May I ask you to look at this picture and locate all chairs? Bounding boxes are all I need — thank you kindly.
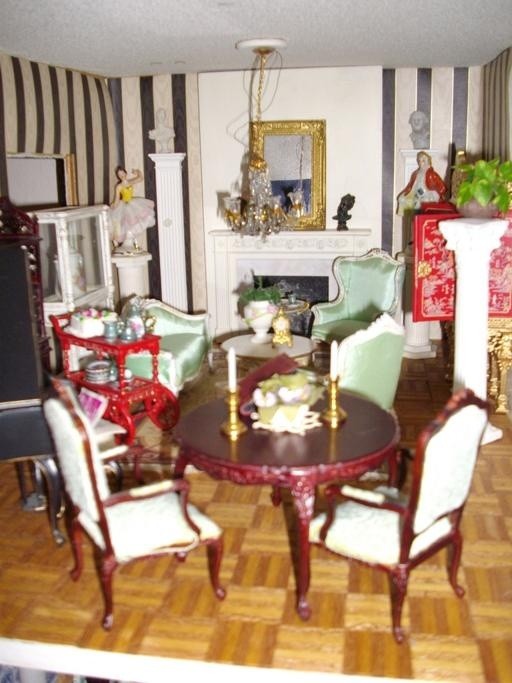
[305,387,490,644]
[39,375,226,630]
[310,247,407,411]
[118,296,218,401]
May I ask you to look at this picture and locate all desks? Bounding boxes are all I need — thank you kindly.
[172,382,402,620]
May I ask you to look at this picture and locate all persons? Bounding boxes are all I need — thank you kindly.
[408,109,431,151]
[394,149,445,213]
[111,164,155,251]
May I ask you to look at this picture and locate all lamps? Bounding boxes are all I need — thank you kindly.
[221,37,304,237]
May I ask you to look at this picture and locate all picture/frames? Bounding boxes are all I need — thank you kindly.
[250,119,327,231]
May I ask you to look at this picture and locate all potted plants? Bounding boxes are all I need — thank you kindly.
[236,274,283,344]
[451,155,512,220]
